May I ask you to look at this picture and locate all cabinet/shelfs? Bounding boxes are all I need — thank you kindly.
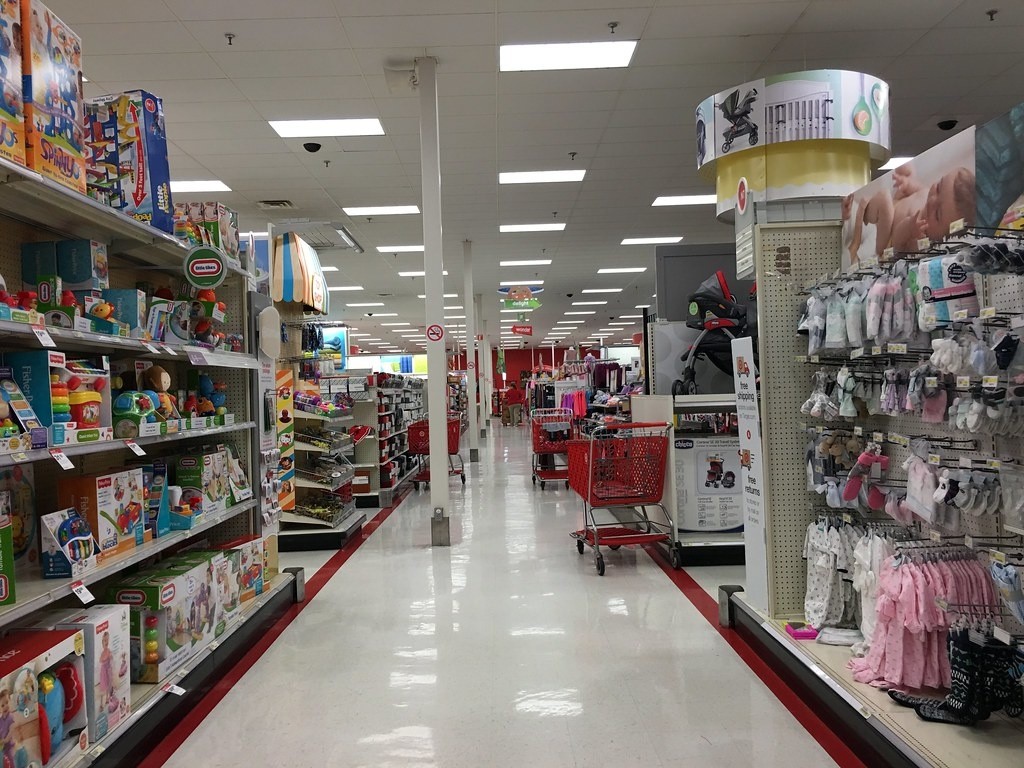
[612,415,632,439]
[376,387,430,508]
[0,157,305,768]
[352,386,381,509]
[278,409,368,553]
[580,403,622,440]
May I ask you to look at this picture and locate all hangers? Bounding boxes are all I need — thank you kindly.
[817,509,1003,639]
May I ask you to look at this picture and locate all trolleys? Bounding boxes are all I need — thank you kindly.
[530,408,574,491]
[565,421,681,576]
[407,411,466,491]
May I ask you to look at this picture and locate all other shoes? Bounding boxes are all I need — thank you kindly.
[511,423,514,427]
[515,424,518,427]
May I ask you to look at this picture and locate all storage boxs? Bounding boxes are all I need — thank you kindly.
[0,629,89,768]
[223,548,245,622]
[144,550,224,655]
[0,0,253,604]
[6,608,85,631]
[104,573,192,685]
[54,604,131,744]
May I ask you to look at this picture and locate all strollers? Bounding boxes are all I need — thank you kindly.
[671,270,759,395]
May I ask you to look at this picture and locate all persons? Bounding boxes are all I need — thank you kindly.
[842,194,877,268]
[52,313,61,326]
[167,606,175,638]
[864,163,974,258]
[584,353,595,360]
[564,347,577,362]
[31,10,50,64]
[0,690,17,761]
[12,23,22,54]
[505,383,522,427]
[98,632,114,711]
[207,568,212,584]
